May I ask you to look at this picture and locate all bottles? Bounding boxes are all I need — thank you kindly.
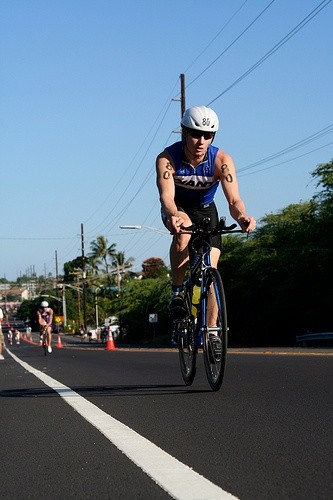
[190,278,202,318]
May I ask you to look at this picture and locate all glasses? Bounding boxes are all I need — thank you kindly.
[185,128,215,140]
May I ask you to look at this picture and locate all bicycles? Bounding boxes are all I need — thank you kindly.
[170,216,247,391]
[41,325,49,356]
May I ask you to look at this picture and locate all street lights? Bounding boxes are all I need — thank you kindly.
[41,289,67,328]
[57,283,104,330]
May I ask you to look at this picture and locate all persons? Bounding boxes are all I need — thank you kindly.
[37,301,54,353]
[156,106,257,363]
[0,309,5,361]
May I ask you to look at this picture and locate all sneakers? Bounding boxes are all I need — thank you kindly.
[208,337,222,363]
[169,296,184,311]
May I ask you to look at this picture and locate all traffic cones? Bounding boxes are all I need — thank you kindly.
[5,320,42,346]
[105,328,118,351]
[56,334,63,348]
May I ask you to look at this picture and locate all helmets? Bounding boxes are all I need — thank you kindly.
[181,106,219,132]
[41,301,48,307]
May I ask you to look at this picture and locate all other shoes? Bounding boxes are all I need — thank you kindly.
[48,346,52,353]
[0,354,4,360]
